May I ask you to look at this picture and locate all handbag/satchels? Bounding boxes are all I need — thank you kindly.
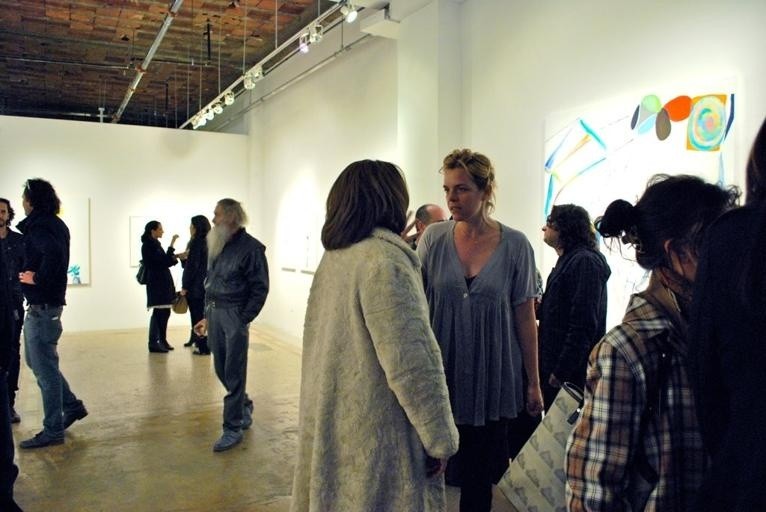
[496,335,673,512]
[135,260,145,284]
[173,292,188,314]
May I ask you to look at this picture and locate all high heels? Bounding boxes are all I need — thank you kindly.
[184,336,198,347]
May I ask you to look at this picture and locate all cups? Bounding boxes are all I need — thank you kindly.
[193,326,207,338]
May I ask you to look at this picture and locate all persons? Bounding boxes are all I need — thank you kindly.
[14,177,88,449]
[0,365,24,511]
[135,220,180,354]
[0,197,26,424]
[192,196,269,453]
[396,201,443,252]
[685,120,765,510]
[173,214,211,356]
[562,172,743,512]
[445,204,611,486]
[287,159,460,512]
[415,146,543,509]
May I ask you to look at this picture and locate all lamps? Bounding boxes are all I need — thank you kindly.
[191,0,358,130]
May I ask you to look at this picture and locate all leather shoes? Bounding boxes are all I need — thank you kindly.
[20,431,63,448]
[213,429,243,451]
[239,404,254,429]
[156,346,168,352]
[62,408,88,430]
[164,344,173,350]
[9,409,20,424]
